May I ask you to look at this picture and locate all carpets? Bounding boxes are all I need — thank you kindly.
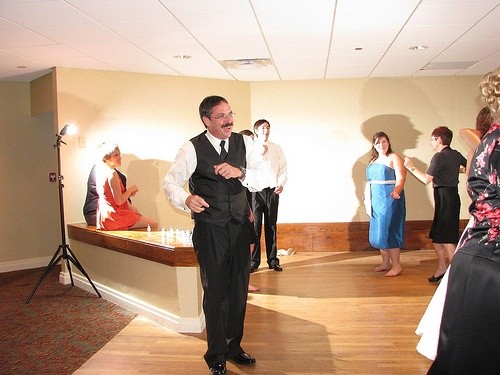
[0,265,139,375]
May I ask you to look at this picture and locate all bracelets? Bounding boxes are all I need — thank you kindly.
[410,167,415,172]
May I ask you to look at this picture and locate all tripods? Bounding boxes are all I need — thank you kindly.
[25,135,102,305]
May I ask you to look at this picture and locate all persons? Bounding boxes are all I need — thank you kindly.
[82,140,159,231]
[415,66,500,375]
[239,118,288,273]
[363,130,408,277]
[164,94,276,375]
[404,126,467,285]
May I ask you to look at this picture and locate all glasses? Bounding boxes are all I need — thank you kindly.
[209,111,237,120]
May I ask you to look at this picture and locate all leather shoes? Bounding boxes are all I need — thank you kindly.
[250,266,258,272]
[269,263,283,271]
[230,352,256,365]
[209,360,227,375]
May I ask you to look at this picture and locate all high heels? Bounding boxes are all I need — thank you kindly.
[428,271,446,283]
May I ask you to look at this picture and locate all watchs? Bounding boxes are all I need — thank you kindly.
[239,166,246,179]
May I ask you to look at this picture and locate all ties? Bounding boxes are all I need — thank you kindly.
[218,140,229,159]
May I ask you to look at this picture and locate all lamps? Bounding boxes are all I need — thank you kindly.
[53,124,69,147]
